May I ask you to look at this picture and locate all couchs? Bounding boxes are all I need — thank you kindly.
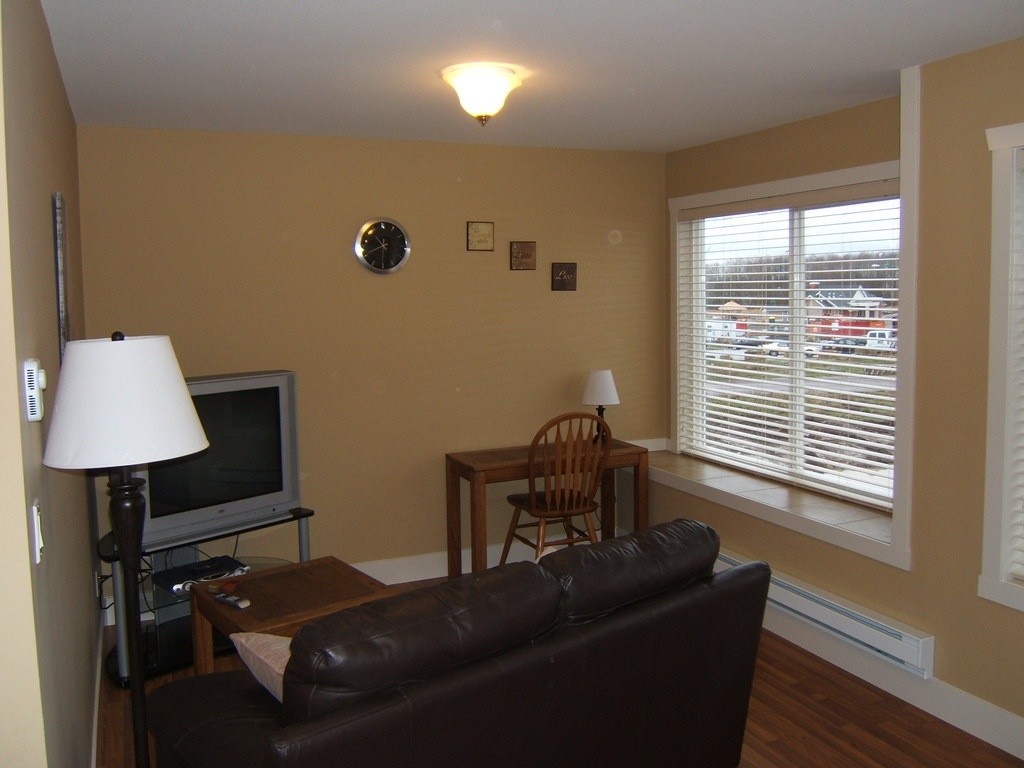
[144,516,770,768]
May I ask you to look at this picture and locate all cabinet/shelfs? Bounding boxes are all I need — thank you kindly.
[97,507,315,689]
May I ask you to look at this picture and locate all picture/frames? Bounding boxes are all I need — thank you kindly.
[551,263,577,292]
[467,220,495,251]
[510,241,536,270]
[55,191,71,364]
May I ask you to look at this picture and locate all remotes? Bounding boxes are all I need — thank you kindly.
[216,592,250,609]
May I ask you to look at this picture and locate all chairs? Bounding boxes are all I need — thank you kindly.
[498,412,612,565]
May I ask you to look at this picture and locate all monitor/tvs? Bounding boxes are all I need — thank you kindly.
[133,368,300,553]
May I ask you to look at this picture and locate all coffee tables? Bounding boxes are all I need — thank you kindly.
[190,555,399,677]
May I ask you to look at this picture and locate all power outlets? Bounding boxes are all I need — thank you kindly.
[94,570,105,597]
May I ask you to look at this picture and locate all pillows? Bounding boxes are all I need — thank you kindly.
[229,632,293,704]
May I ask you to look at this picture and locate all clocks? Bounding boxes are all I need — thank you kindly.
[354,217,412,274]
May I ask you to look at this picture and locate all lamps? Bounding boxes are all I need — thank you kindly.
[43,330,210,768]
[439,61,523,127]
[581,369,621,444]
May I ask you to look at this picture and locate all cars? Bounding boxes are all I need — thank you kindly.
[763,341,819,357]
[728,339,763,351]
[821,338,867,354]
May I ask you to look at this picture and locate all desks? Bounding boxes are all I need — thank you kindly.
[445,438,649,583]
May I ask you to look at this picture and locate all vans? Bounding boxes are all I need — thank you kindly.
[867,330,893,348]
